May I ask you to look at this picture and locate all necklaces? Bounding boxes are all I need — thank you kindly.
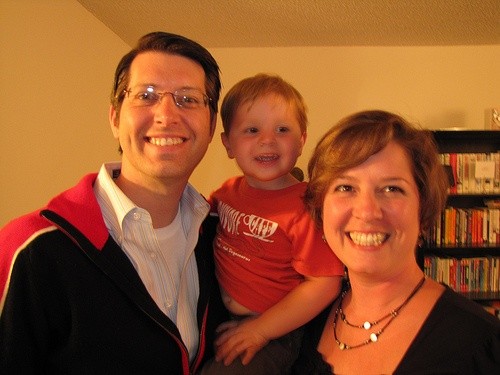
[333,272,428,351]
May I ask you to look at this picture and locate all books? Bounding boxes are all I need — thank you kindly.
[423,201,500,245]
[437,152,499,194]
[423,254,499,293]
[484,302,500,317]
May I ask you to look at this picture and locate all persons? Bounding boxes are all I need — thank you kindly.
[290,110,500,375]
[0,30,224,375]
[198,73,344,375]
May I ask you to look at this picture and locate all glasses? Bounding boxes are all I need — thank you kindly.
[123,84,212,112]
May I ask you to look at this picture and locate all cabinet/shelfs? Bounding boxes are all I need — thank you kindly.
[413,129,500,320]
